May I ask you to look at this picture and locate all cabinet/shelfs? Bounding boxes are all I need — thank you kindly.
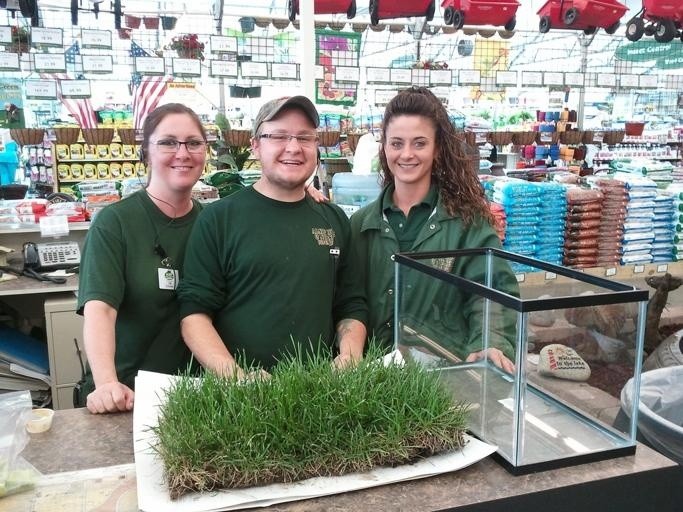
[42,296,87,410]
[56,159,142,184]
[593,138,683,172]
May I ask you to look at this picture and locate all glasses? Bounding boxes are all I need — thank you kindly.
[257,134,318,146]
[144,138,206,153]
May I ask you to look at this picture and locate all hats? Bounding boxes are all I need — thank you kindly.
[254,95,320,135]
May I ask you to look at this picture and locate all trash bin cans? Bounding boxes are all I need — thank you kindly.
[612,365,683,466]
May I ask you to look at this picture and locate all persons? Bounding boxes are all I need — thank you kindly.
[176,95,367,390]
[349,84,519,379]
[75,101,329,416]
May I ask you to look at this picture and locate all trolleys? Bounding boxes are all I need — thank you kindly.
[437,0,522,31]
[365,0,436,28]
[535,1,629,36]
[286,0,357,22]
[624,1,682,45]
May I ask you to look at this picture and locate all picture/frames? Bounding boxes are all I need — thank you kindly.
[1,26,114,101]
[313,64,480,85]
[136,34,298,80]
[496,70,586,87]
[596,72,658,88]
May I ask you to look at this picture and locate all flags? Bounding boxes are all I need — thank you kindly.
[36,40,98,129]
[130,37,175,134]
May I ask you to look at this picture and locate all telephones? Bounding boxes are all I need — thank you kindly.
[22,241,82,272]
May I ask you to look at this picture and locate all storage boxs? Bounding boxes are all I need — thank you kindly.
[331,173,382,213]
[391,247,648,475]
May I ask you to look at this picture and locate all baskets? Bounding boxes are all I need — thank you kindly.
[486,131,626,145]
[117,128,144,144]
[456,132,476,146]
[346,133,365,155]
[221,129,252,147]
[10,128,115,145]
[318,131,341,147]
